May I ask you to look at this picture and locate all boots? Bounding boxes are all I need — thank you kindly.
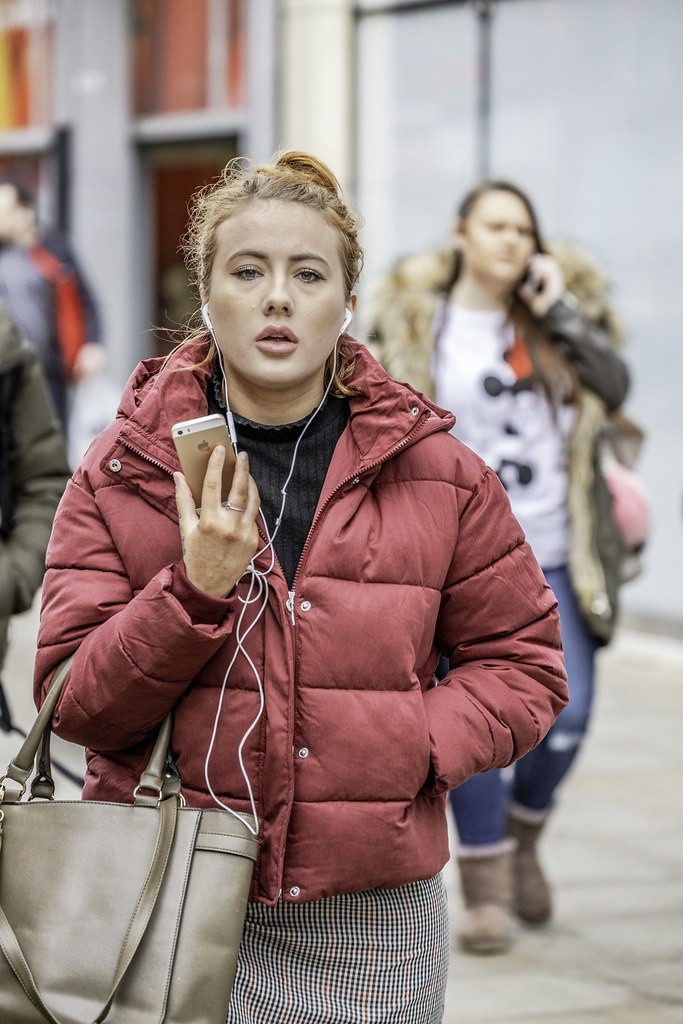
[456,835,518,950]
[505,796,554,923]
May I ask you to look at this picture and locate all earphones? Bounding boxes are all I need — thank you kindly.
[340,307,352,333]
[201,302,212,330]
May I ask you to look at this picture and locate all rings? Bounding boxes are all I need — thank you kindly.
[226,501,245,512]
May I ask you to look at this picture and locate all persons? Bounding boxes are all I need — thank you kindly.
[0,177,109,672]
[364,180,631,957]
[33,148,569,1024]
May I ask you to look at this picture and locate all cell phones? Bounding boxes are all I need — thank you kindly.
[524,268,541,291]
[171,413,238,516]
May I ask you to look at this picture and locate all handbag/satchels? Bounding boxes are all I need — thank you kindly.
[594,438,652,550]
[0,658,263,1024]
